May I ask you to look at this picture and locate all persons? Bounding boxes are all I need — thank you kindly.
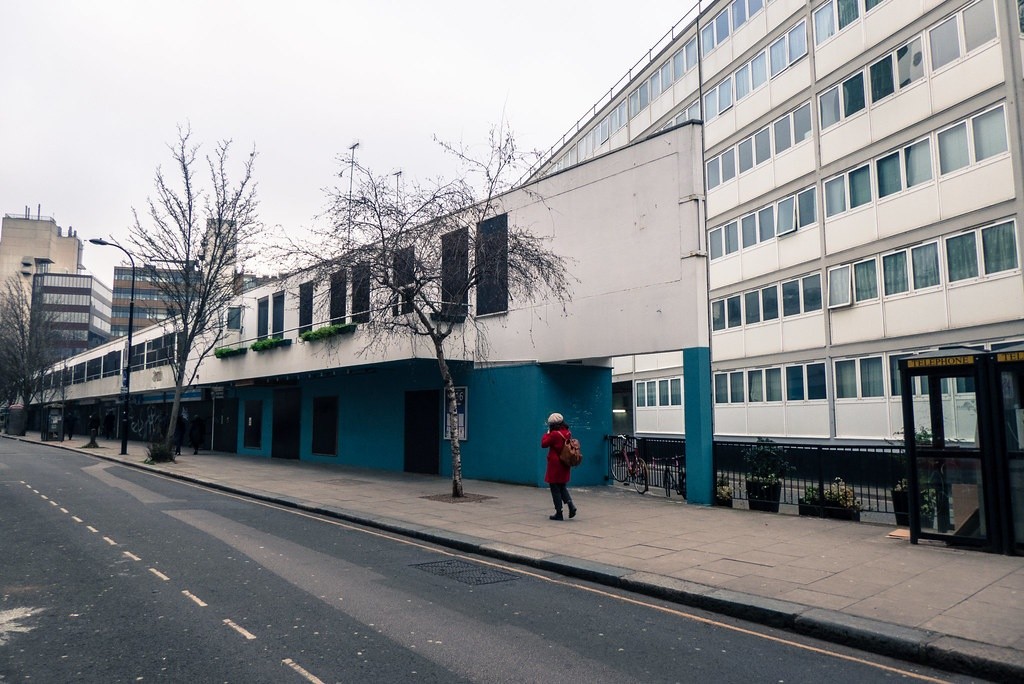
[64,409,205,457]
[540,412,576,520]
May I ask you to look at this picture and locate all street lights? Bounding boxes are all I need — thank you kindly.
[90,238,136,455]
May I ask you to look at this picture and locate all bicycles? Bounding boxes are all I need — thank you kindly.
[609,434,649,495]
[653,455,687,500]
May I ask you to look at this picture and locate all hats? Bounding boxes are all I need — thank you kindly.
[548,413,563,423]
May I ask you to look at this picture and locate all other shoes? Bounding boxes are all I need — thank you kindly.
[549,502,577,521]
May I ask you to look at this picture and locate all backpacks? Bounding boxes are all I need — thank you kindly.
[557,431,582,466]
